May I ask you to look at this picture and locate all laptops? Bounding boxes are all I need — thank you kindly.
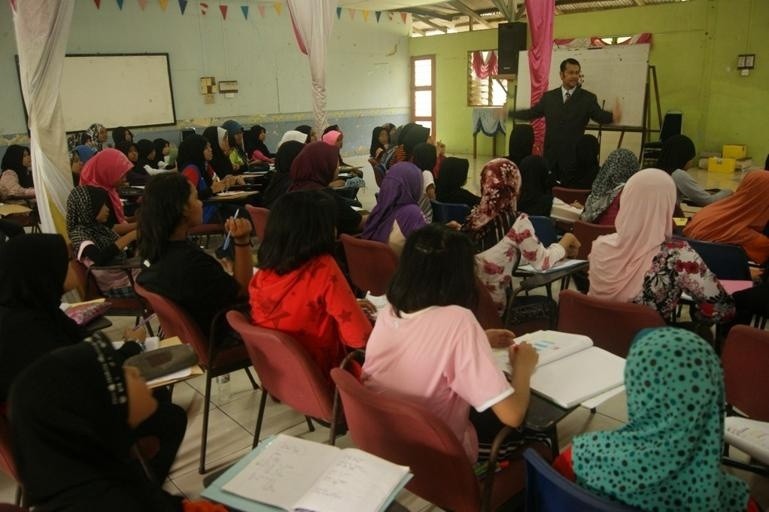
[643,111,682,148]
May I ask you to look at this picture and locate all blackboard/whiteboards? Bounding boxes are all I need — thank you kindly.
[514,43,651,132]
[14,53,177,138]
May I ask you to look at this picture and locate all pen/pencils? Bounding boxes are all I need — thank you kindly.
[222,208,240,251]
[122,313,157,338]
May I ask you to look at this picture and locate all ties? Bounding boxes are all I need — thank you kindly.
[566,92,571,100]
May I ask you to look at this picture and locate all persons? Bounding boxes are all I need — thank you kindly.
[493,58,623,182]
[2,120,769,510]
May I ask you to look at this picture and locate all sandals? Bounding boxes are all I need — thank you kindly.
[212,253,233,275]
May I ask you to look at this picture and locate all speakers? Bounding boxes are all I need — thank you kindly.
[497,22,527,74]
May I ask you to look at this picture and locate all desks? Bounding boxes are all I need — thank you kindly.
[472,106,506,159]
